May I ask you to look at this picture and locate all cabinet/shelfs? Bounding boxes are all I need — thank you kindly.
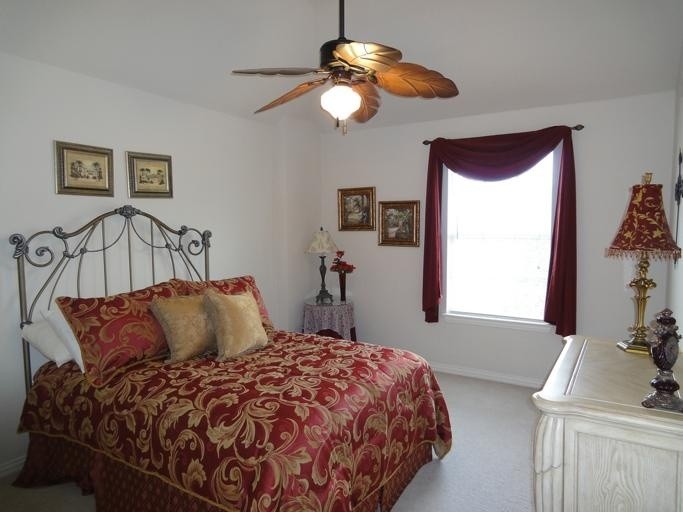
[531,335,682,511]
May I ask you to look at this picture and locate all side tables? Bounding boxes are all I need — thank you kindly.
[302,295,356,343]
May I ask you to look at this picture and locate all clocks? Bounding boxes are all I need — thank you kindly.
[642,308,683,413]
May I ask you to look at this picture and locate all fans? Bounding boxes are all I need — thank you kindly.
[231,0,460,123]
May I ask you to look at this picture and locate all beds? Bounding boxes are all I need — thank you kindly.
[9,205,452,512]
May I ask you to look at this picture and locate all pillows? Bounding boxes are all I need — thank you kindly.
[21,320,72,368]
[42,304,85,375]
[171,275,272,327]
[55,281,181,390]
[148,294,218,364]
[202,287,268,363]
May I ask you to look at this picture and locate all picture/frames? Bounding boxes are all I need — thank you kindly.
[338,187,377,232]
[125,150,173,199]
[377,200,420,247]
[53,139,115,198]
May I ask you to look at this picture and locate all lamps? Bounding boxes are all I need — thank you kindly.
[304,226,340,304]
[607,171,682,355]
[319,73,361,136]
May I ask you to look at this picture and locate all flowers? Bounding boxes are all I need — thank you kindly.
[330,250,354,273]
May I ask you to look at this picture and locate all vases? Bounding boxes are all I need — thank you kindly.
[339,273,346,301]
